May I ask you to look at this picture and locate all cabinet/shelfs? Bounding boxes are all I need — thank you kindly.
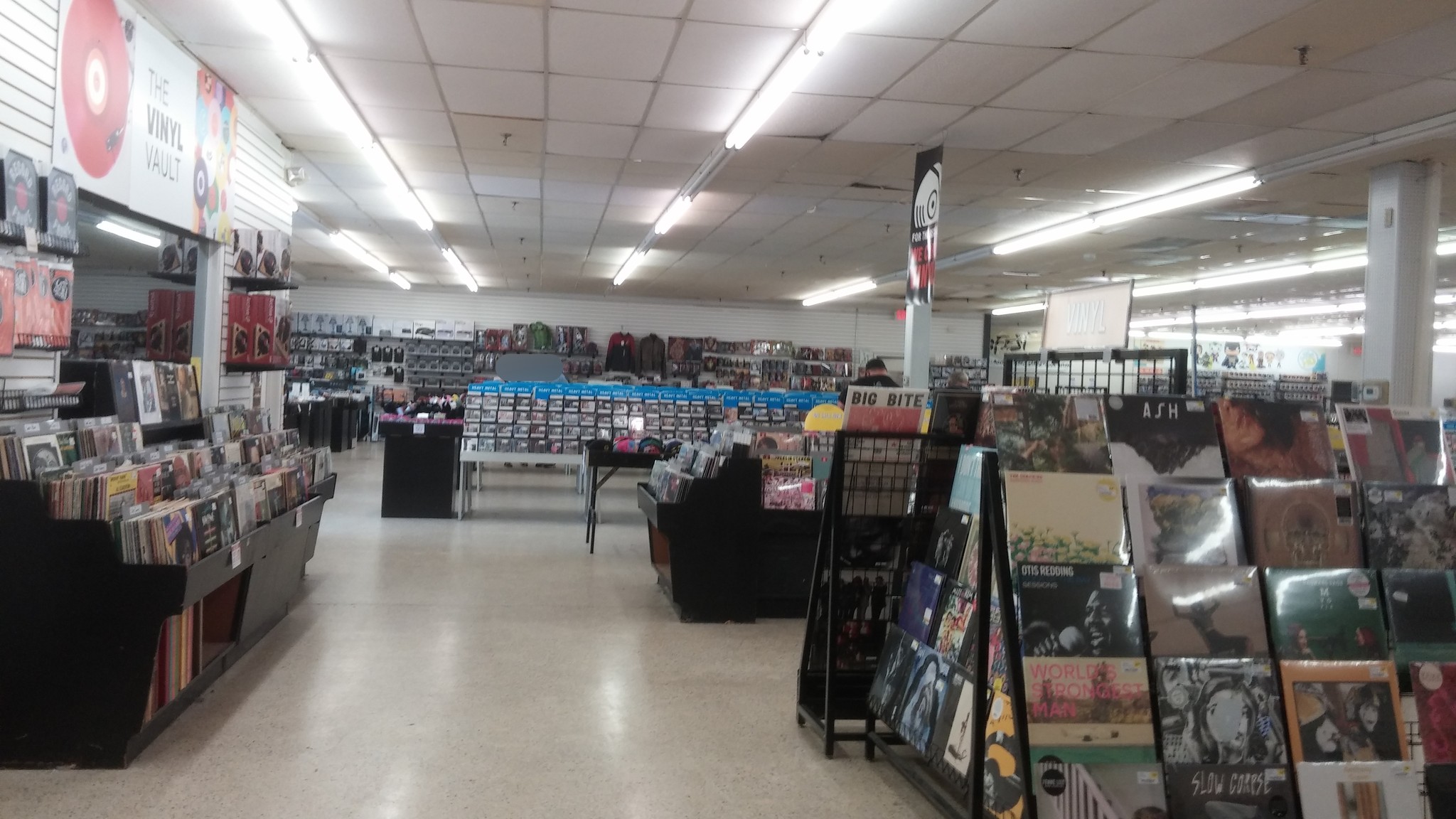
[0,278,1456,819]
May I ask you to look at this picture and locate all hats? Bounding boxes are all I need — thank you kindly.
[585,436,684,454]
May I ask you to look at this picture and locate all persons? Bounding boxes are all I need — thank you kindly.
[946,371,969,389]
[838,358,899,410]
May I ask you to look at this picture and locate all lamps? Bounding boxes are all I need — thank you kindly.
[97,0,1266,309]
